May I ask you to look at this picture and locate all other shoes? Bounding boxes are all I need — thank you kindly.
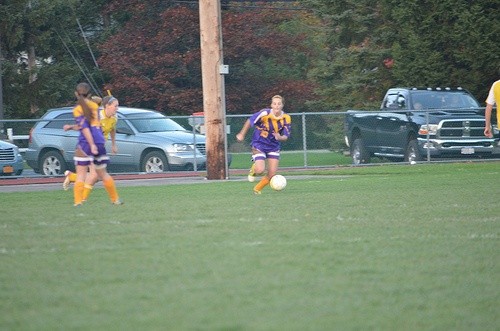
[248,163,256,182]
[253,187,261,195]
[62,170,71,190]
[112,200,124,206]
[73,200,87,208]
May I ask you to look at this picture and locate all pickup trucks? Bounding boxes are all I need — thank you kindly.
[343,85,500,165]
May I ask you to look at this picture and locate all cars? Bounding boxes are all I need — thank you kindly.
[0,140,25,179]
[25,106,232,174]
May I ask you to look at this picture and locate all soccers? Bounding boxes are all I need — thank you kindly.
[269,173,288,191]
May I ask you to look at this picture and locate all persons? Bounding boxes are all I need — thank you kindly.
[63,83,124,207]
[484,80,500,140]
[236,95,292,194]
